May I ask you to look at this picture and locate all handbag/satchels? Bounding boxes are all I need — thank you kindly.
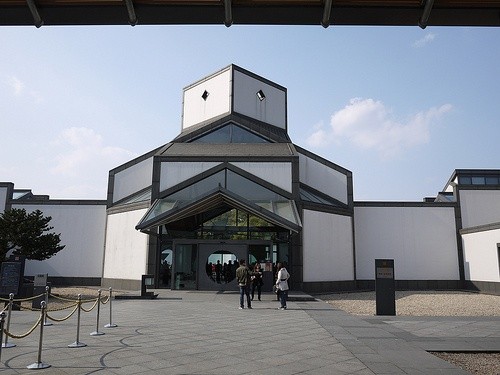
[258,277,264,286]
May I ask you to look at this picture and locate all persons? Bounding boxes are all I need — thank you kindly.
[250,262,263,302]
[206,260,239,283]
[236,259,262,310]
[273,262,290,311]
